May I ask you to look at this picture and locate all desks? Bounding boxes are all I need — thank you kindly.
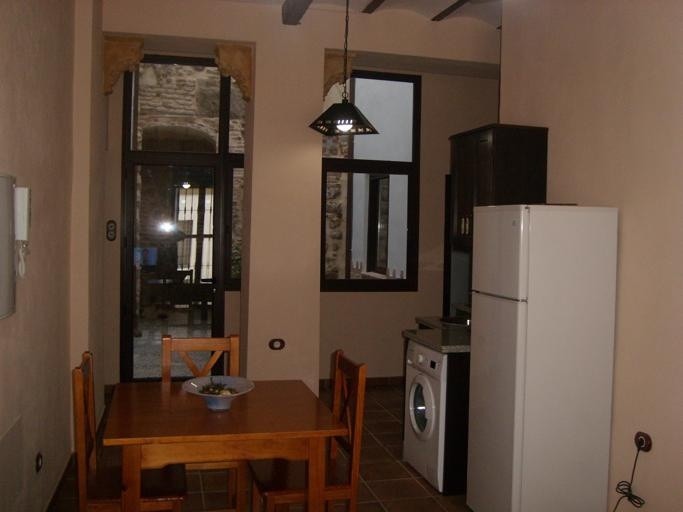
[149,278,212,305]
[100,378,349,512]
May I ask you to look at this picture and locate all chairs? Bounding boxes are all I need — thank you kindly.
[251,348,367,512]
[71,351,186,510]
[163,269,193,311]
[158,335,242,512]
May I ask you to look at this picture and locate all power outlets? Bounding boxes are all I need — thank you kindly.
[635,432,652,452]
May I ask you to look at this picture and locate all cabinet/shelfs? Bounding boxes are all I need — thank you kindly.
[448,124,549,239]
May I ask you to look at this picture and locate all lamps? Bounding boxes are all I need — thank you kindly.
[182,182,191,189]
[309,1,379,136]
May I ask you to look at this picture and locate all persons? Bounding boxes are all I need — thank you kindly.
[141,250,153,265]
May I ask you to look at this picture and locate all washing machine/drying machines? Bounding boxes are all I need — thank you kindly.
[401,339,471,496]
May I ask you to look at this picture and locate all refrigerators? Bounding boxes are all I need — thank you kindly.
[465,204,621,511]
